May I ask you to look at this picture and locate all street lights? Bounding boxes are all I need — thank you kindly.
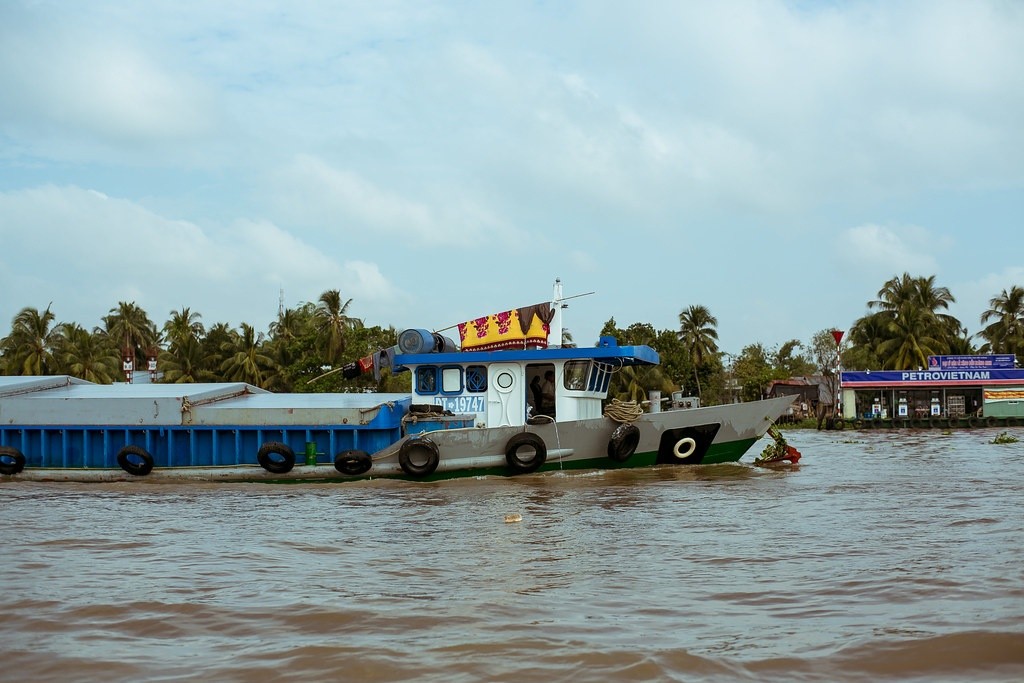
[830,331,845,418]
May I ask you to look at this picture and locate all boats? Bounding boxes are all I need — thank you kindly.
[0,276,801,482]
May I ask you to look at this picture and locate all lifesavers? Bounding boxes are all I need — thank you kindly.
[852,418,884,429]
[505,432,547,474]
[947,416,960,428]
[116,445,153,476]
[607,423,641,463]
[909,417,922,429]
[1005,416,1019,427]
[334,449,372,475]
[929,417,943,429]
[891,417,904,428]
[831,416,846,430]
[399,436,439,478]
[0,447,25,474]
[257,441,295,474]
[967,417,979,429]
[985,416,1000,428]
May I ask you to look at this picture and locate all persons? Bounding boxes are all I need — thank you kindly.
[540,370,555,411]
